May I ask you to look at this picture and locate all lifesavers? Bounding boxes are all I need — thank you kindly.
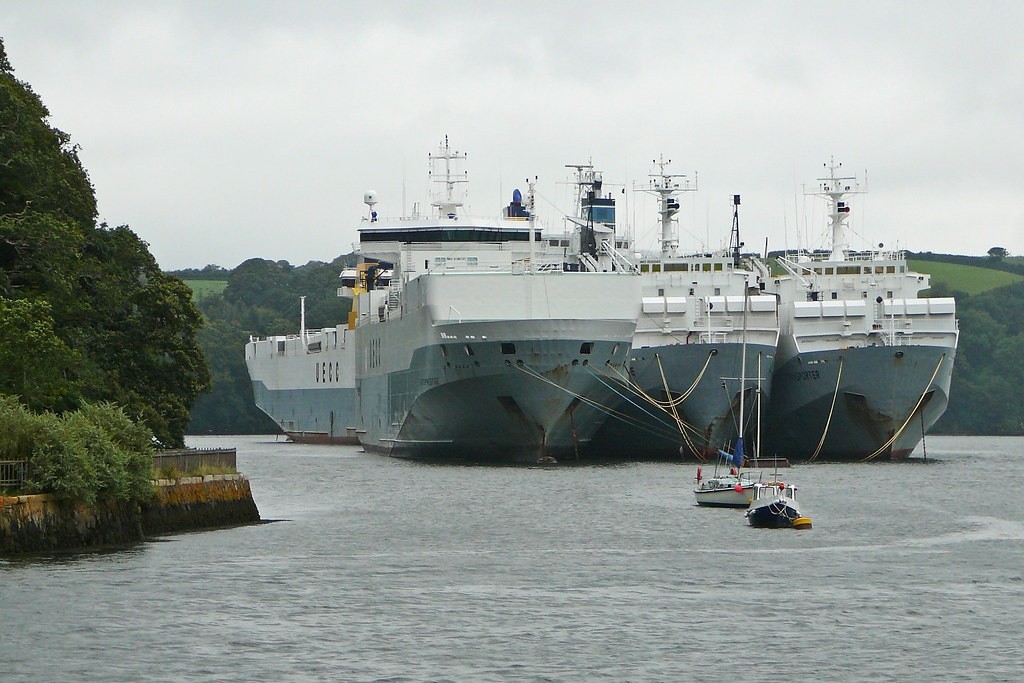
[731,468,736,475]
[697,466,701,480]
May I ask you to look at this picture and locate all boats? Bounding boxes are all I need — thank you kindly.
[745,452,812,530]
[245,130,960,465]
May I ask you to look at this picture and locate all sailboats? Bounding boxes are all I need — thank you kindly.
[692,280,800,510]
